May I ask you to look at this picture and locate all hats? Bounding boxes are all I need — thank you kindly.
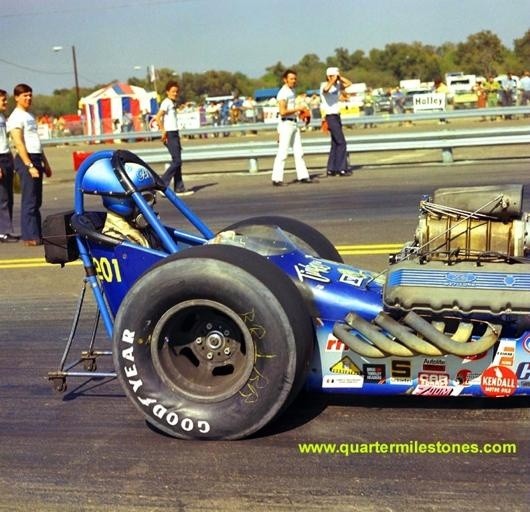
[327,67,339,75]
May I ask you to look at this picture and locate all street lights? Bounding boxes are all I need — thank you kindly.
[134,66,156,98]
[52,45,80,114]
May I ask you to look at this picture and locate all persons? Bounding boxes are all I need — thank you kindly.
[1,88,21,240]
[270,68,318,188]
[321,64,354,177]
[6,84,52,247]
[159,82,194,198]
[44,66,529,146]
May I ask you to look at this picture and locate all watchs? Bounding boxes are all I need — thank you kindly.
[26,162,34,169]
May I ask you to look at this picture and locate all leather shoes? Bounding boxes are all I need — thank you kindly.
[1,233,43,246]
[327,170,336,177]
[340,169,351,174]
[273,181,288,185]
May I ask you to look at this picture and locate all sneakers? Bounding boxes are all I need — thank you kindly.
[176,189,193,196]
[298,179,312,183]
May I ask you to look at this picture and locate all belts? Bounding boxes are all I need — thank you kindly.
[282,117,297,122]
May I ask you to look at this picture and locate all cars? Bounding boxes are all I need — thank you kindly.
[41,149,530,441]
